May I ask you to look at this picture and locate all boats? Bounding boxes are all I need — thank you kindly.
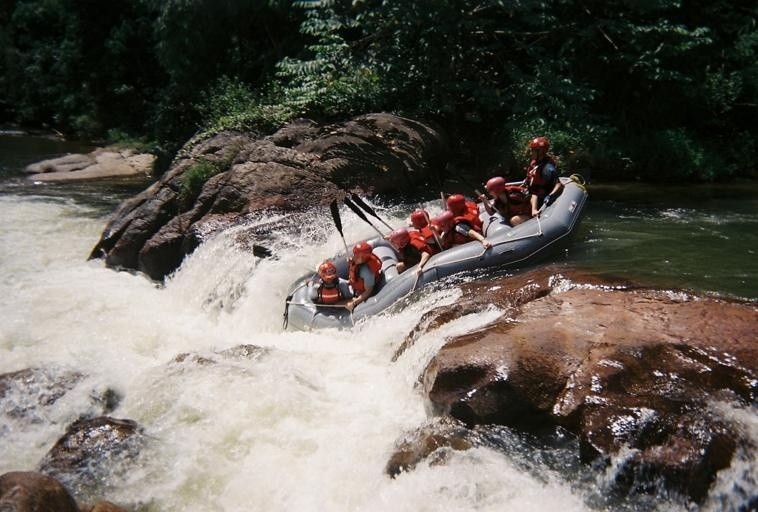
[284,175,588,335]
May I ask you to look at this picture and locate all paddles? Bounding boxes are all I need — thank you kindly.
[344,196,385,239]
[456,172,503,215]
[403,167,443,251]
[330,199,351,268]
[253,245,318,273]
[351,193,394,231]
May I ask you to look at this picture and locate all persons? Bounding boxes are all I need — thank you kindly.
[427,210,492,250]
[346,242,382,312]
[444,194,483,236]
[308,262,354,311]
[478,177,532,227]
[408,209,440,253]
[384,228,434,276]
[519,137,564,206]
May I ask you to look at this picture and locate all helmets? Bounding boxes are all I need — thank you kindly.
[389,229,409,248]
[447,194,465,209]
[320,263,337,282]
[528,137,548,153]
[353,243,372,262]
[411,211,429,226]
[487,176,505,193]
